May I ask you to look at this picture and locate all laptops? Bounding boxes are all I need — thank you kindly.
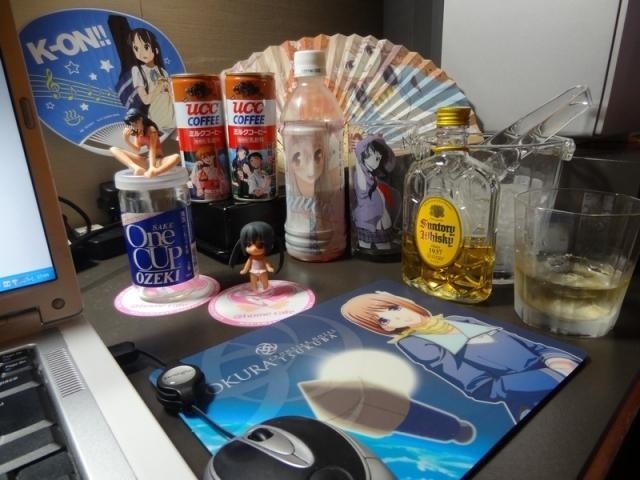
[0,0,198,480]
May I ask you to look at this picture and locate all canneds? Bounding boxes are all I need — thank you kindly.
[224,71,277,202]
[170,73,231,203]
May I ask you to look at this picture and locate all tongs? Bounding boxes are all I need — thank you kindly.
[480,82,595,184]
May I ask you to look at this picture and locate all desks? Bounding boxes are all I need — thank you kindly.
[76,250,640,479]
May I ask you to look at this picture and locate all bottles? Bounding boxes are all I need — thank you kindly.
[280,50,351,262]
[402,105,494,303]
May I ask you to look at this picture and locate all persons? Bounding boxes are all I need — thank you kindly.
[227,220,286,291]
[109,109,178,178]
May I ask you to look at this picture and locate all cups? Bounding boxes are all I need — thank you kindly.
[514,189,640,337]
[412,128,576,285]
[347,121,417,254]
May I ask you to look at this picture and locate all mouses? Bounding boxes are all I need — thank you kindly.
[203,416,396,480]
